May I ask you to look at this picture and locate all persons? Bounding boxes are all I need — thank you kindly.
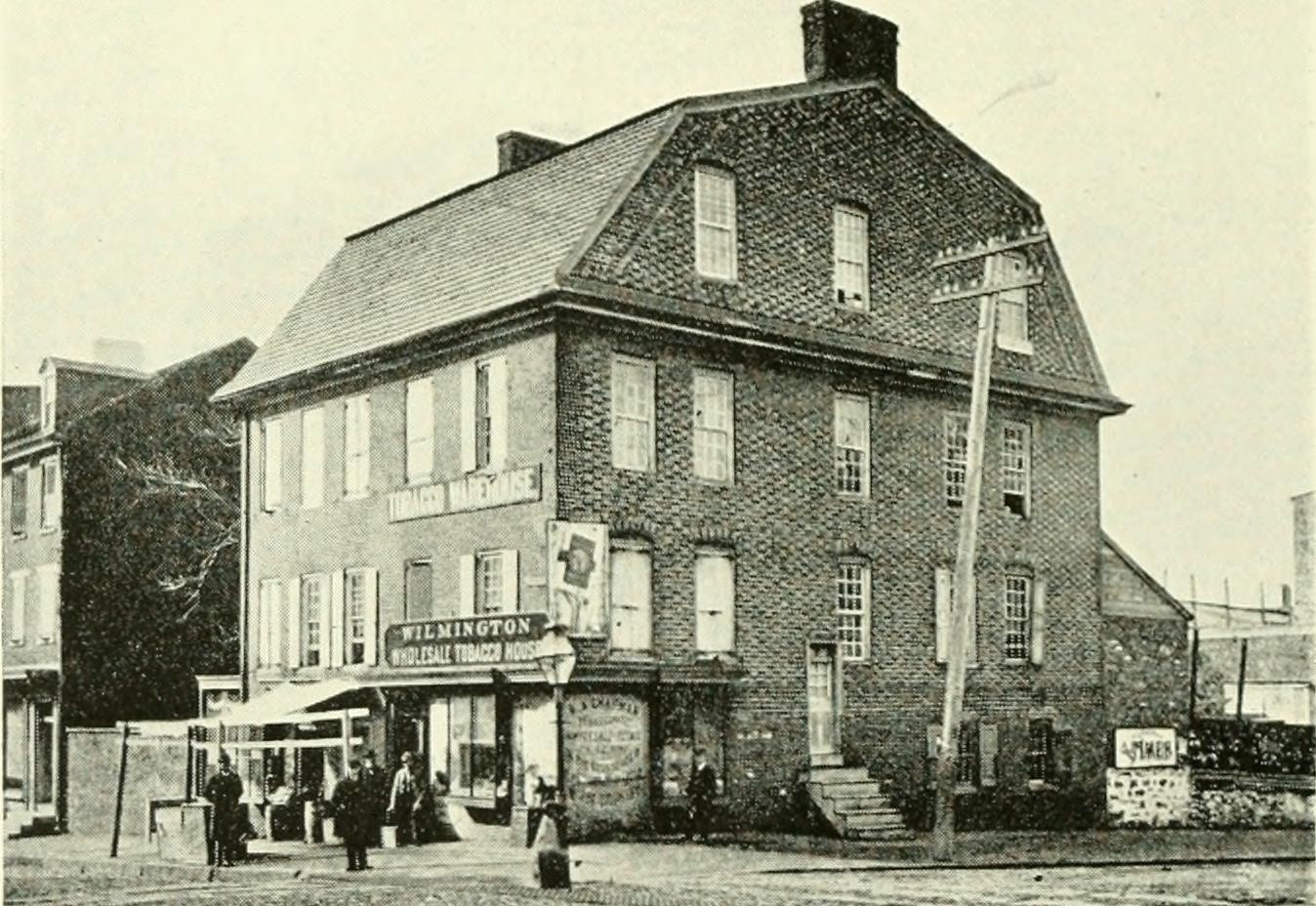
[252,750,414,868]
[678,748,716,837]
[201,756,242,865]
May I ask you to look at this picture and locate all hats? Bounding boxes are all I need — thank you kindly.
[218,754,230,763]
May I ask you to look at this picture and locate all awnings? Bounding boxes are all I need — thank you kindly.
[214,683,364,723]
